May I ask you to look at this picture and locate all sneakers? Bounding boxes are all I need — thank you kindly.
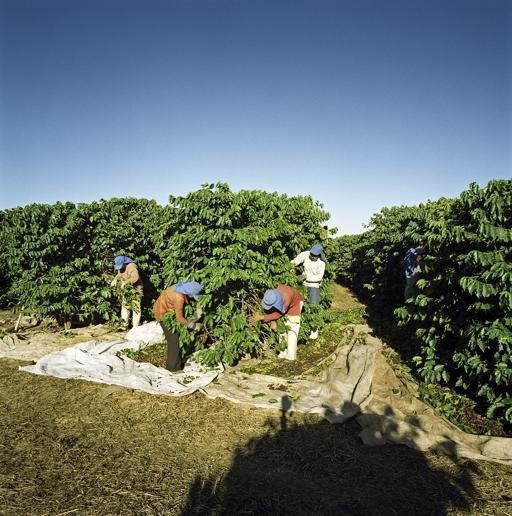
[309,328,318,339]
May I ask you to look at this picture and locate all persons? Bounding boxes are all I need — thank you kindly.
[153,280,203,371]
[289,245,326,340]
[248,283,304,360]
[110,255,144,331]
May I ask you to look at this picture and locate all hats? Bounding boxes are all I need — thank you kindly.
[310,244,327,263]
[114,256,134,270]
[261,289,286,313]
[403,248,417,261]
[174,279,203,301]
